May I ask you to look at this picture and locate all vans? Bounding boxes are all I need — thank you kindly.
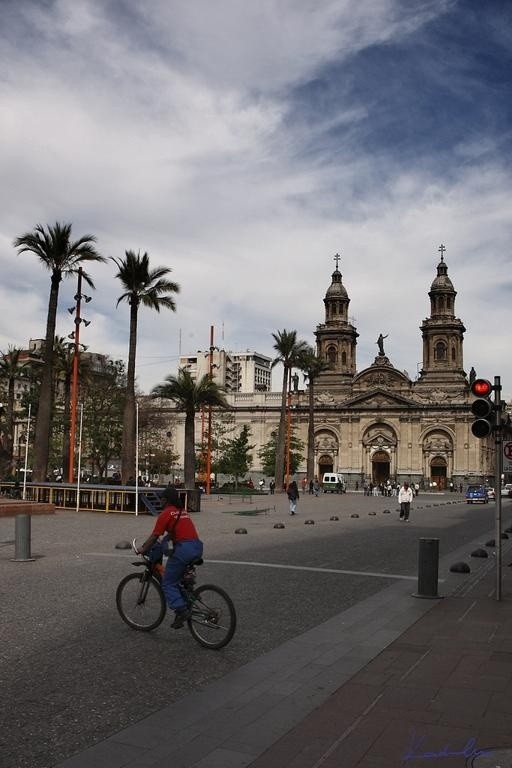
[322,472,347,494]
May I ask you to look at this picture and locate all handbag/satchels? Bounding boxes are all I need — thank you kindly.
[150,540,169,564]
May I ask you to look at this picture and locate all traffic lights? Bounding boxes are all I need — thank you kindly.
[470,378,492,440]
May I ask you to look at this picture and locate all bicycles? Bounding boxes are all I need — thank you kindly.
[115,537,237,651]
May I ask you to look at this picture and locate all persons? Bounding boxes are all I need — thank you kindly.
[397,481,413,523]
[56,471,181,487]
[137,486,203,628]
[10,481,21,500]
[291,372,302,391]
[377,333,388,353]
[356,478,464,497]
[469,366,477,384]
[259,479,322,515]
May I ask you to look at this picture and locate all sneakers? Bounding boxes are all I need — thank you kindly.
[170,608,192,629]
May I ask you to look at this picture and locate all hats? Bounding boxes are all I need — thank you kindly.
[159,488,182,507]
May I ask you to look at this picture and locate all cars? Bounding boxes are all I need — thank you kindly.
[464,483,512,504]
